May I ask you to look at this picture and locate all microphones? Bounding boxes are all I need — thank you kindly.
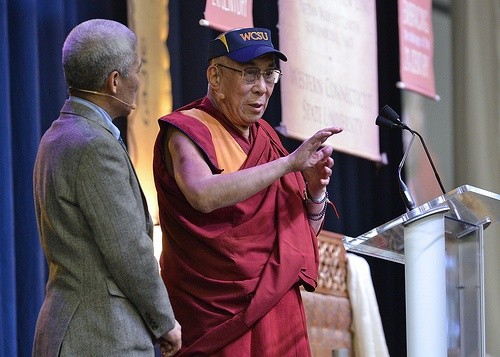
[375,115,446,194]
[380,104,416,211]
[67,85,137,110]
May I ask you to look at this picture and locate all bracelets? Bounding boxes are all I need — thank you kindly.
[305,181,341,221]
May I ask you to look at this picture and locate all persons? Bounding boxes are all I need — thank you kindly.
[32,18,181,357]
[153,27,344,357]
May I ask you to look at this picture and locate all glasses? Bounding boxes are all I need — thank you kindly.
[217,63,283,84]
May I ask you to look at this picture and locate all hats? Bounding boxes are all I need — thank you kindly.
[208,27,287,64]
